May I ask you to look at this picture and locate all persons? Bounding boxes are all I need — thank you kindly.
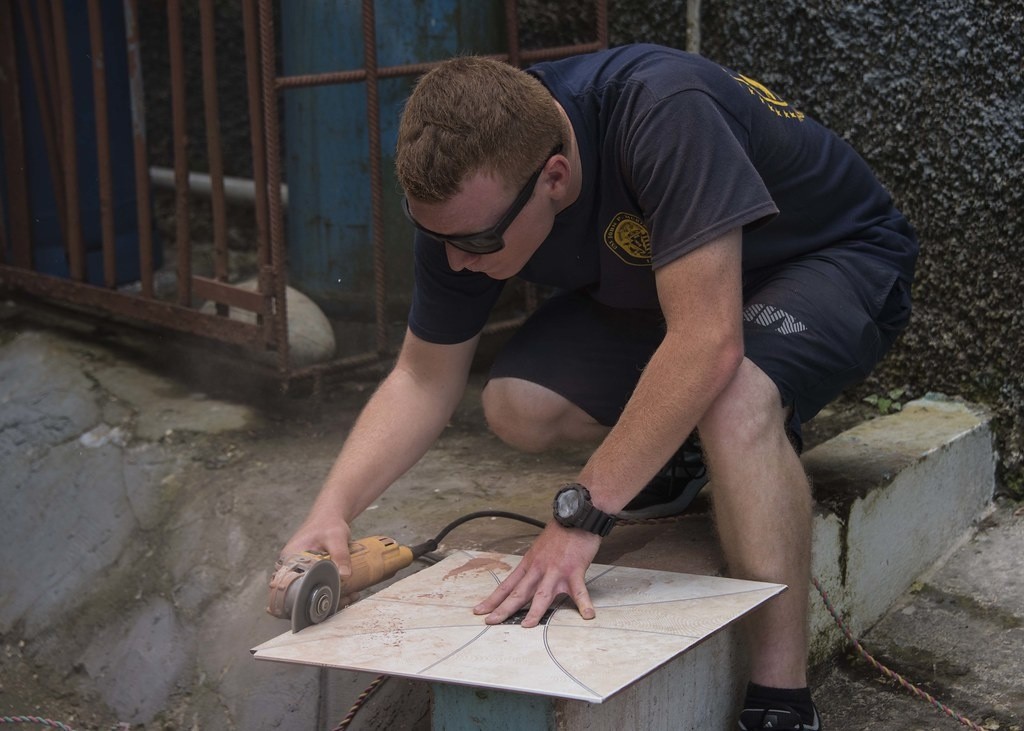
[280,44,919,730]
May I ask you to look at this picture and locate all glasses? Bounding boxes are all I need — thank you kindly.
[400,142,563,255]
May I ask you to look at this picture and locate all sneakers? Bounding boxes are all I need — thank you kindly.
[734,696,821,731]
[615,410,805,520]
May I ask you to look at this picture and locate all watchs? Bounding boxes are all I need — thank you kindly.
[554,482,615,539]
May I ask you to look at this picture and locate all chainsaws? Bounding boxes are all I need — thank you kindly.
[265,536,438,634]
